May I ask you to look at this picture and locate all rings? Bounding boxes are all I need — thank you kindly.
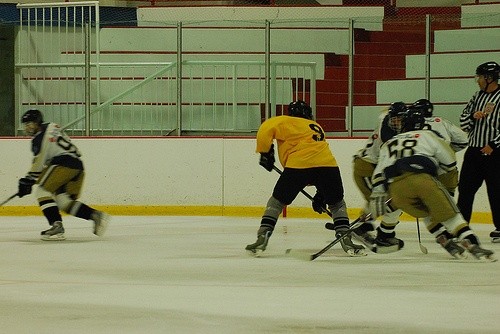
[484,153,487,155]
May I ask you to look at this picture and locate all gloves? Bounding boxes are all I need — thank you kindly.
[259,144,275,172]
[18,176,35,198]
[366,193,387,219]
[312,192,327,215]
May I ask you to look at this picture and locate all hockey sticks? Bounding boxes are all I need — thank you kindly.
[416,217,428,255]
[284,195,393,261]
[273,166,401,254]
[0,193,19,206]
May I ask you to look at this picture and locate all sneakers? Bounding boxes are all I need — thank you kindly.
[335,231,367,256]
[40,222,65,241]
[351,222,376,253]
[443,239,468,259]
[245,227,274,257]
[372,227,404,254]
[468,244,497,263]
[92,210,112,238]
[490,227,500,243]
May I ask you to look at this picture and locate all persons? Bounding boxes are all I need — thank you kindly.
[17,109,109,239]
[243,99,367,257]
[352,62,500,263]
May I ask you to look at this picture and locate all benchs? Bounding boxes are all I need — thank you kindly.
[20,5,500,136]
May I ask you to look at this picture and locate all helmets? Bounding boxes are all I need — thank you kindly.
[20,110,44,125]
[401,111,424,134]
[288,100,312,119]
[408,99,434,117]
[475,61,500,79]
[388,102,408,119]
